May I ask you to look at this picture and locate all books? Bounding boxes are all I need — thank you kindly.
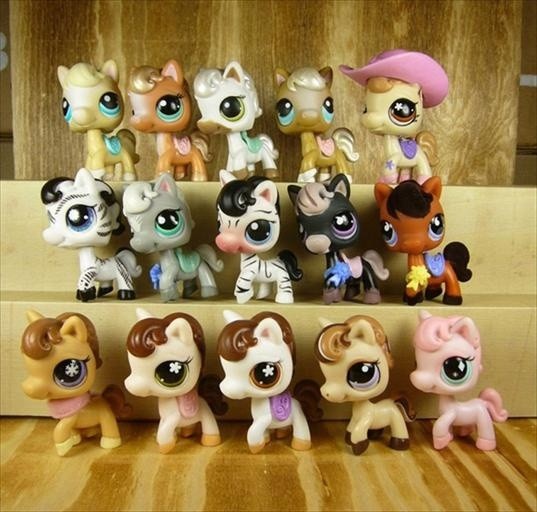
[18,306,133,458]
[285,172,391,305]
